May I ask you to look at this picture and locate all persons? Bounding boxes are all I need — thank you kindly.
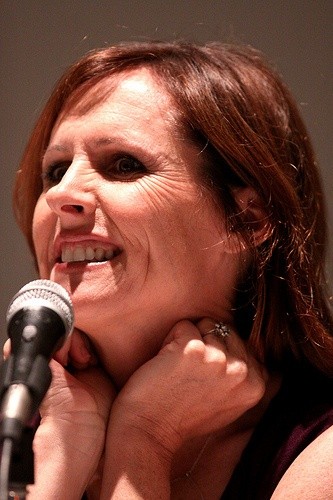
[0,39,333,500]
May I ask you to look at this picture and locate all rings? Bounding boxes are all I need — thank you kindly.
[201,320,232,339]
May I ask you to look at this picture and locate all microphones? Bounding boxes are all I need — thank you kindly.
[0,278,76,445]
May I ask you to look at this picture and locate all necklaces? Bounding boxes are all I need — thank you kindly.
[171,432,212,483]
